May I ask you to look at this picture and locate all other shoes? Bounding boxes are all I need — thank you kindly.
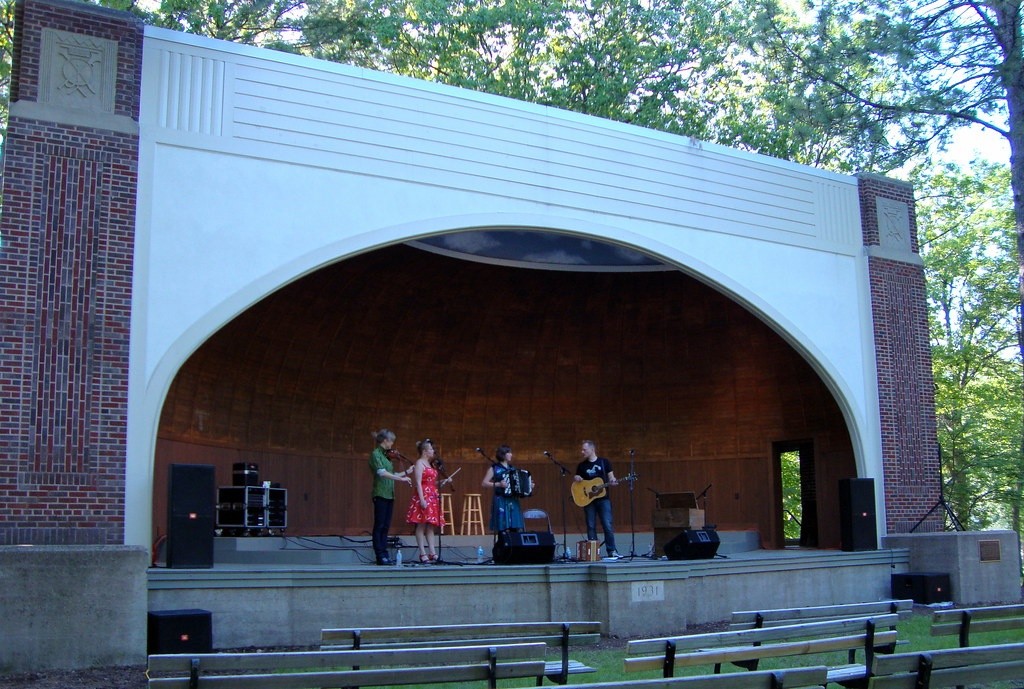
[608,551,623,559]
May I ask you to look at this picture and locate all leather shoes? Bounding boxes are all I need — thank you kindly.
[386,558,396,565]
[378,557,393,565]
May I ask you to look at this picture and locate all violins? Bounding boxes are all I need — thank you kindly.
[431,454,457,492]
[386,448,415,471]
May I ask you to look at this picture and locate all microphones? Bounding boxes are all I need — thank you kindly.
[544,452,552,456]
[476,448,480,451]
[629,450,635,455]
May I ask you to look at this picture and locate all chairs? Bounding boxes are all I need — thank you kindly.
[522,508,551,534]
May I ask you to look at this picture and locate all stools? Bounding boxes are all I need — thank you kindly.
[460,493,486,536]
[439,493,456,536]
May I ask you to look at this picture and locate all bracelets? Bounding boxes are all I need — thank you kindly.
[404,469,407,475]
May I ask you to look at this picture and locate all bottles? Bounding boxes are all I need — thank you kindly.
[648,542,653,557]
[396,550,402,566]
[565,547,571,562]
[477,545,484,563]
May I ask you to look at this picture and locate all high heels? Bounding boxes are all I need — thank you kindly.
[419,554,432,564]
[428,553,438,561]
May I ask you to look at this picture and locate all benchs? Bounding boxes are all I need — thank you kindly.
[148,599,1024,689]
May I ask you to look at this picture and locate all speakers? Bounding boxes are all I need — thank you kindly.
[891,573,950,604]
[165,464,215,567]
[663,530,721,559]
[147,609,212,653]
[838,478,877,551]
[491,532,556,565]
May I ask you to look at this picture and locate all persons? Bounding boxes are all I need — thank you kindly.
[368,429,415,565]
[482,445,535,541]
[574,439,624,559]
[405,440,453,564]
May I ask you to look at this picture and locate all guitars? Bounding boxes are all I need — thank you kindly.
[570,473,638,506]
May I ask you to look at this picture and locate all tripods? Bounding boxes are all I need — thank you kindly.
[618,455,651,560]
[909,443,967,532]
[426,470,465,566]
[547,454,581,563]
